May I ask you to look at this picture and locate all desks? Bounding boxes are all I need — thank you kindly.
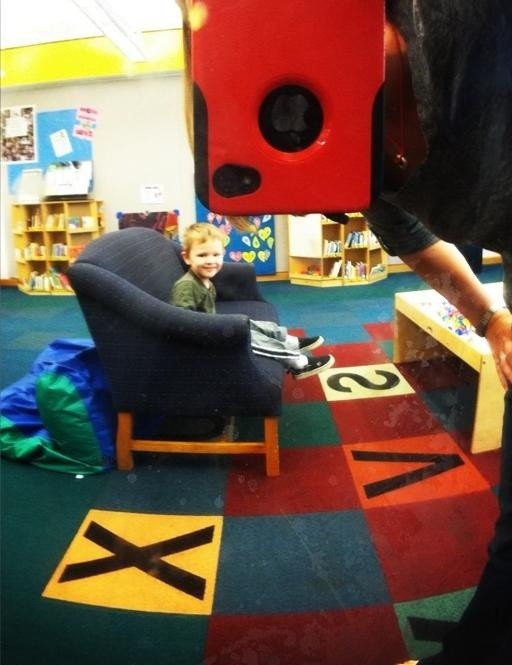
[392,281,511,455]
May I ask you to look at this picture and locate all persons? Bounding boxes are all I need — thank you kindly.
[169,219,337,384]
[357,0,512,664]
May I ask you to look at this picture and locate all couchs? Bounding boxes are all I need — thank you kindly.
[65,225,285,478]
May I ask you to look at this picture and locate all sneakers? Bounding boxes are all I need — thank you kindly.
[293,355,335,380]
[299,336,324,353]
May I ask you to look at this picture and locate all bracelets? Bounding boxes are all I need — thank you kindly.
[473,304,500,338]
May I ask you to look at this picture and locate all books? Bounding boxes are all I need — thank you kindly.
[21,211,95,292]
[323,228,386,282]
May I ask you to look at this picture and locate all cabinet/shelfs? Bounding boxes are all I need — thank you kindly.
[288,208,388,288]
[11,199,107,296]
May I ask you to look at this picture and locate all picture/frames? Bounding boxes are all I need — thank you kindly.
[0,104,39,165]
